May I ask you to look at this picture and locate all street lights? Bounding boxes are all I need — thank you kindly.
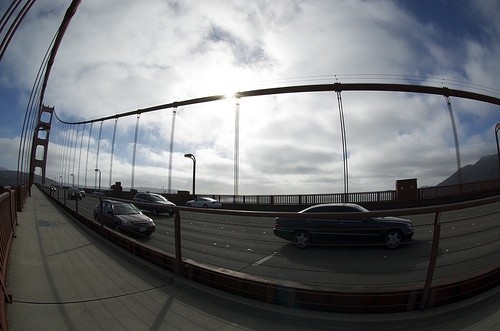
[94,169,101,192]
[71,174,75,188]
[184,153,196,200]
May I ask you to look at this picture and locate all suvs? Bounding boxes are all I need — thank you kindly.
[131,193,176,217]
[68,188,86,200]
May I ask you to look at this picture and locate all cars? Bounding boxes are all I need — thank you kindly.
[185,196,222,209]
[272,203,415,250]
[93,199,156,239]
[41,184,56,191]
